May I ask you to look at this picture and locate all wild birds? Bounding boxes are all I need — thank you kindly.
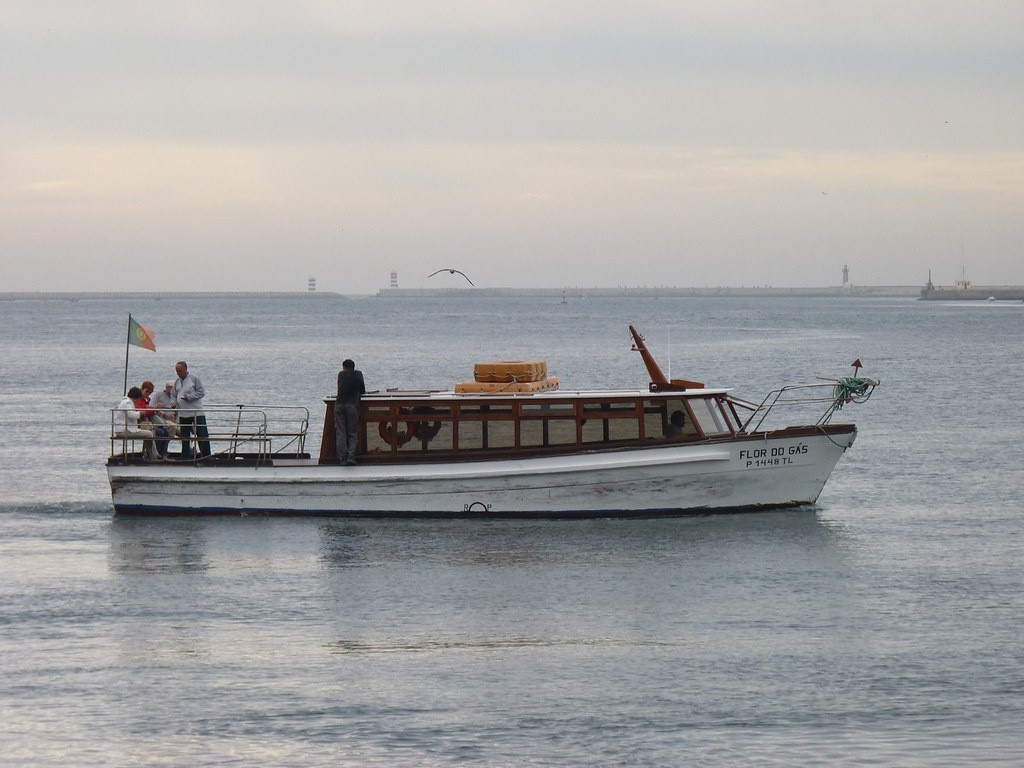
[426,268,475,287]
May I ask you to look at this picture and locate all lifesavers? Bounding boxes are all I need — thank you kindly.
[406,406,442,442]
[378,408,415,446]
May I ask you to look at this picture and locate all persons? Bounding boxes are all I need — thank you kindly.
[114,379,180,460]
[332,358,366,464]
[666,410,686,437]
[170,361,211,459]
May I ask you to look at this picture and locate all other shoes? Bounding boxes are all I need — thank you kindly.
[143,454,162,460]
[346,458,358,466]
[341,459,347,466]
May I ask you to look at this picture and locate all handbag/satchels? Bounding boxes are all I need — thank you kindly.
[139,420,153,433]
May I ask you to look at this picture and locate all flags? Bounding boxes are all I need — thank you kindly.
[129,316,157,353]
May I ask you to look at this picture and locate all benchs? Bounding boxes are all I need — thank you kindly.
[204,432,308,459]
[109,435,272,466]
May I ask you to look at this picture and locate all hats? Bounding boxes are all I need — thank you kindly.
[166,382,176,387]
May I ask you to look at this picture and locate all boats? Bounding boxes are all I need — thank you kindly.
[106,313,881,519]
[917,267,1024,301]
